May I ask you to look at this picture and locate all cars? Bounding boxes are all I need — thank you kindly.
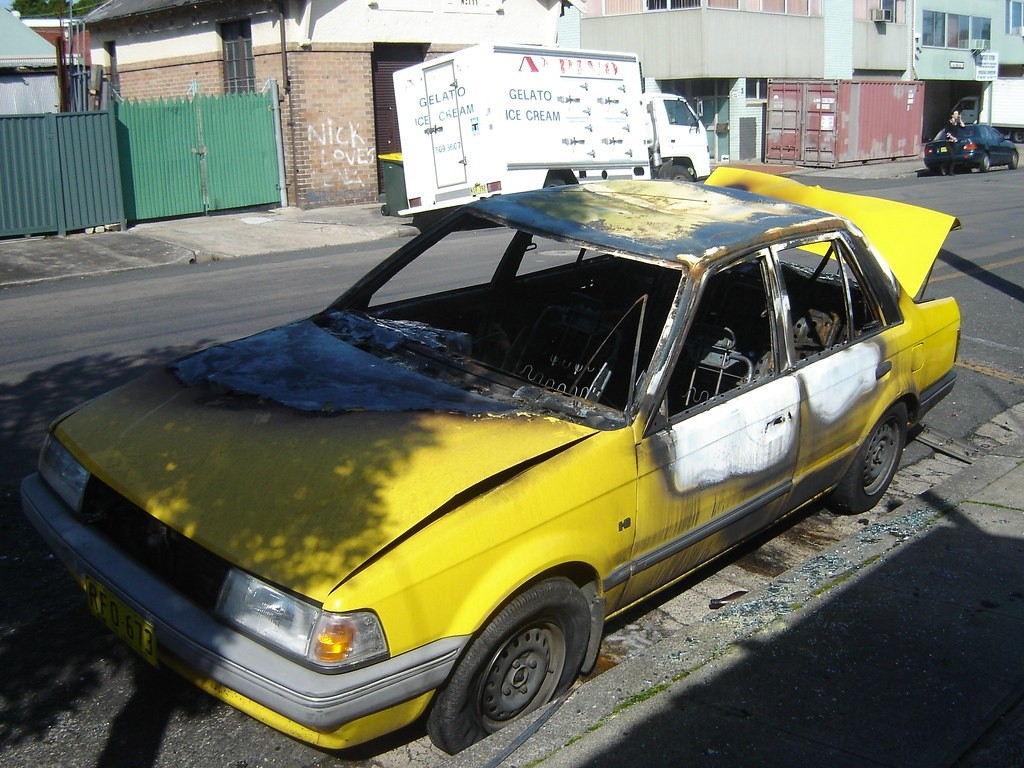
[924,125,1019,174]
[19,165,962,755]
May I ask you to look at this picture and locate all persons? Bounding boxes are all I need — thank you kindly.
[939,109,965,177]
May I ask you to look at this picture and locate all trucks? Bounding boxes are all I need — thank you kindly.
[947,76,1024,143]
[392,46,710,236]
